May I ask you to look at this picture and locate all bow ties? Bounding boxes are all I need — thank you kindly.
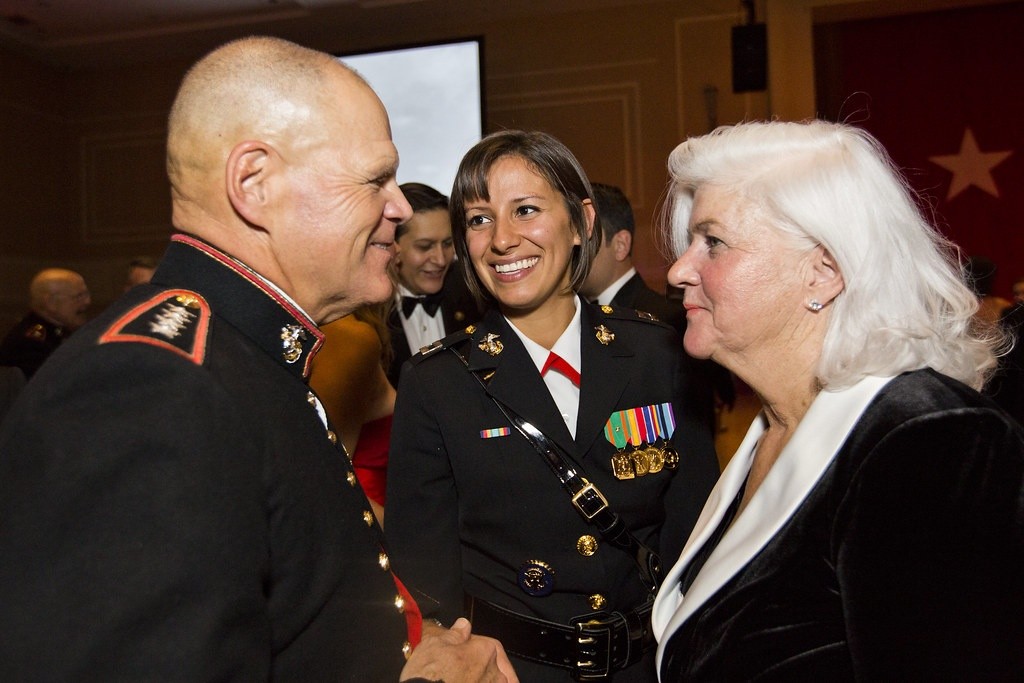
[402,294,442,319]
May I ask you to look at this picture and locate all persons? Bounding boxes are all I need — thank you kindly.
[1,266,94,385]
[651,122,1024,683]
[310,243,400,524]
[382,129,721,683]
[577,183,738,409]
[353,182,483,386]
[0,32,521,683]
[957,252,1024,418]
